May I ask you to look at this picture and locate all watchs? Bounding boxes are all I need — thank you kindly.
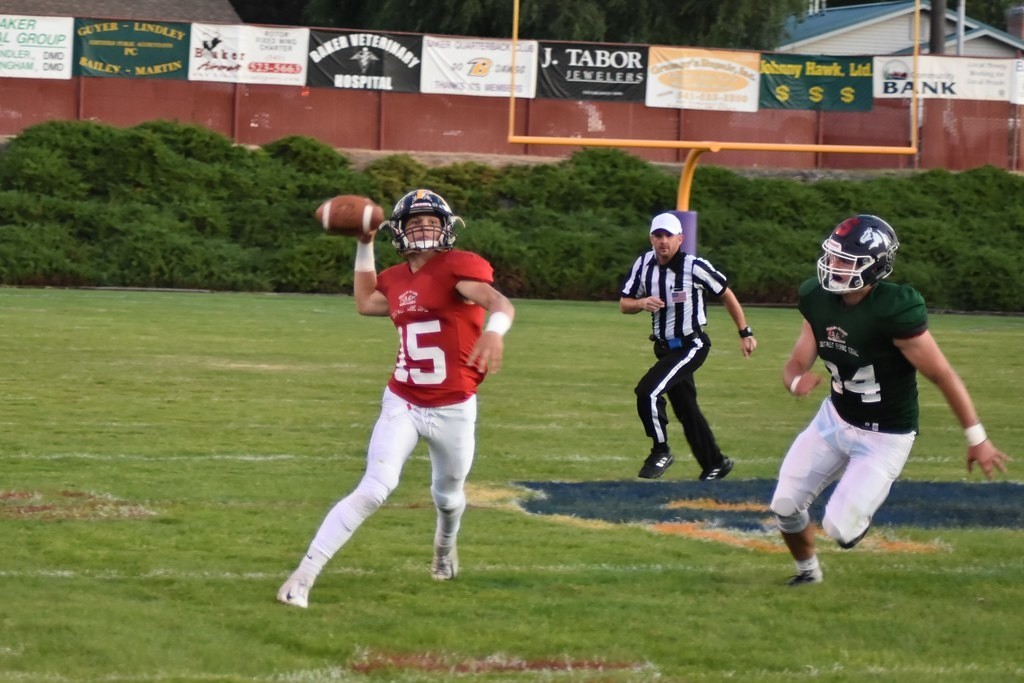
[739,326,752,337]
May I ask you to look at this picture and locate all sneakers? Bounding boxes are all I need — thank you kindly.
[431,543,458,580]
[788,567,823,585]
[837,526,870,549]
[278,577,309,608]
[639,447,674,478]
[700,456,734,481]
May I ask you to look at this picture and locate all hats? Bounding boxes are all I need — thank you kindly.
[650,212,682,235]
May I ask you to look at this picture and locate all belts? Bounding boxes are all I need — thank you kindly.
[657,331,698,345]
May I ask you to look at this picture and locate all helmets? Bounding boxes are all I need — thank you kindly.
[829,214,898,288]
[389,189,453,254]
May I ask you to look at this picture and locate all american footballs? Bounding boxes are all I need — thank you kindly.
[313,195,385,234]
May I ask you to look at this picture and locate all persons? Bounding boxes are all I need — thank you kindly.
[769,215,1012,589]
[619,212,759,480]
[274,190,514,608]
